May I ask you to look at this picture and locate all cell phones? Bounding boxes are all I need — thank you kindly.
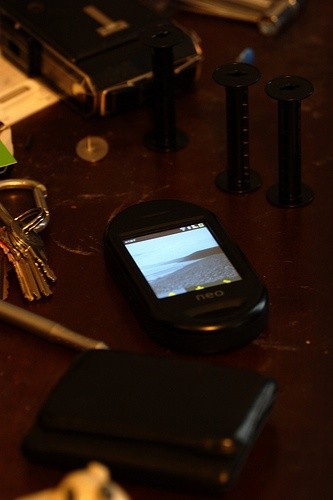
[105,197,271,354]
[19,349,280,499]
[0,0,203,120]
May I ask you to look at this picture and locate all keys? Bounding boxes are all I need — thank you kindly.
[0,178,59,304]
